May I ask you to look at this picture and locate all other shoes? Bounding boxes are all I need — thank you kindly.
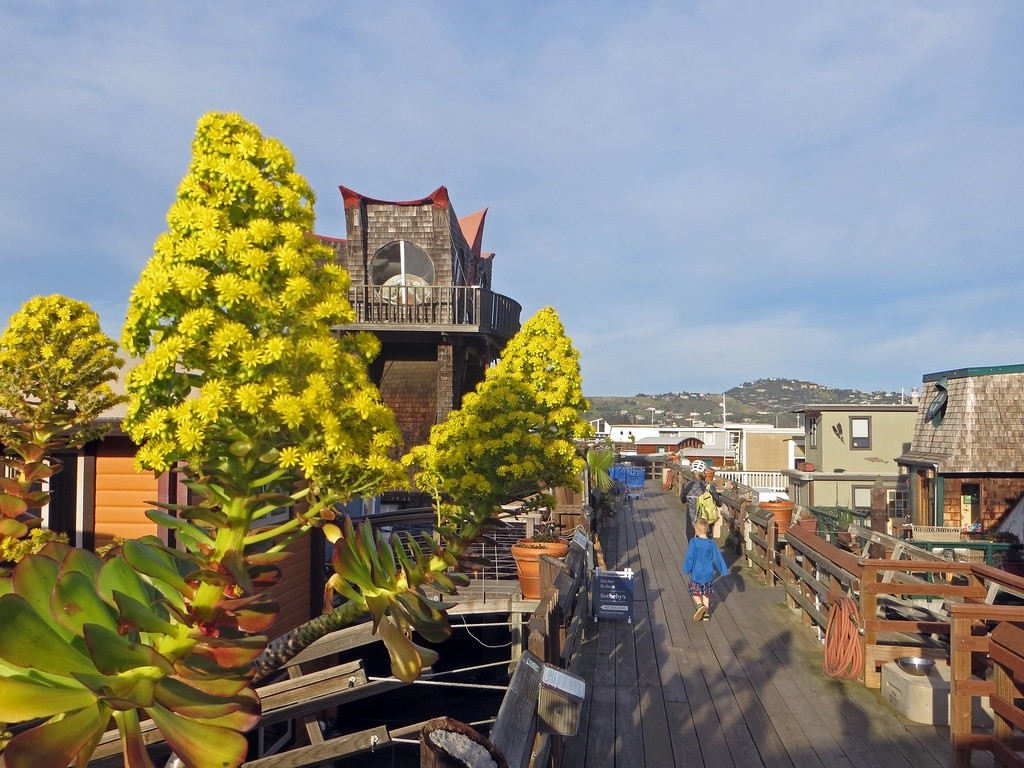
[702,613,709,621]
[693,603,706,621]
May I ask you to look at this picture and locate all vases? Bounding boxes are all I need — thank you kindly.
[758,500,796,538]
[511,543,568,599]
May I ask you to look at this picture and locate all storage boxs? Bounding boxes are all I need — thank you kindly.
[536,662,587,738]
[879,663,994,726]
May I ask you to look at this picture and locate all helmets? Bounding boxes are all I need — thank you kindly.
[690,460,707,473]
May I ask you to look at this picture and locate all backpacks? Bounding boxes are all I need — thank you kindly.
[694,484,718,525]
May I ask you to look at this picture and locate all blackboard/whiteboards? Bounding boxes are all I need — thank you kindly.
[592,576,634,619]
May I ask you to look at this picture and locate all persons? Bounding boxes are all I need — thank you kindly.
[680,459,722,546]
[684,518,729,621]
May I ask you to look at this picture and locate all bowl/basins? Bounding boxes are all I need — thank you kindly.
[898,658,935,676]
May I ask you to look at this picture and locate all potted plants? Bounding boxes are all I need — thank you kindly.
[516,529,568,545]
[798,510,817,530]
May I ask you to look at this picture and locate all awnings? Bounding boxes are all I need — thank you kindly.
[894,451,954,467]
